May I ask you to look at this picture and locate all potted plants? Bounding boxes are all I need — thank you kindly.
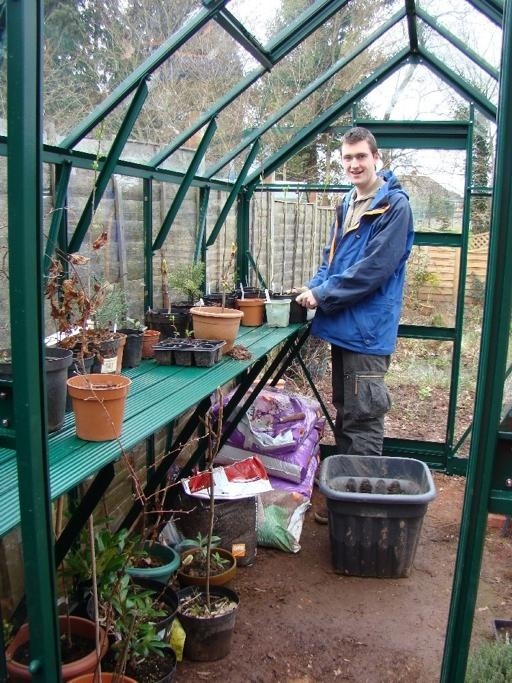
[177,535,237,587]
[418,270,443,316]
[76,366,209,597]
[74,271,127,375]
[174,384,239,659]
[225,271,239,308]
[104,584,178,683]
[171,261,205,338]
[404,268,427,309]
[46,109,184,586]
[58,497,179,669]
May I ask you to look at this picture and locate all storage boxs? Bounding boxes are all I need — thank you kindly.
[320,456,436,580]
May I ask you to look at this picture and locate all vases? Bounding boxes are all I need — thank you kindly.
[235,298,265,326]
[109,333,127,376]
[0,347,72,431]
[189,308,243,354]
[242,292,266,323]
[69,353,96,413]
[117,328,143,369]
[205,294,227,306]
[148,308,185,341]
[153,338,226,366]
[66,328,118,376]
[273,293,307,323]
[239,287,263,292]
[194,497,257,563]
[141,330,160,359]
[6,616,109,683]
[68,374,132,442]
[264,299,291,327]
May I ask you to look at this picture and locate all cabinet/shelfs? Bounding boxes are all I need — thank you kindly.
[0,321,341,683]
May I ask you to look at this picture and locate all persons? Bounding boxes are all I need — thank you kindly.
[285,126,413,525]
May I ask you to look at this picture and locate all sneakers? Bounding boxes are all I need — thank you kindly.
[315,507,330,525]
